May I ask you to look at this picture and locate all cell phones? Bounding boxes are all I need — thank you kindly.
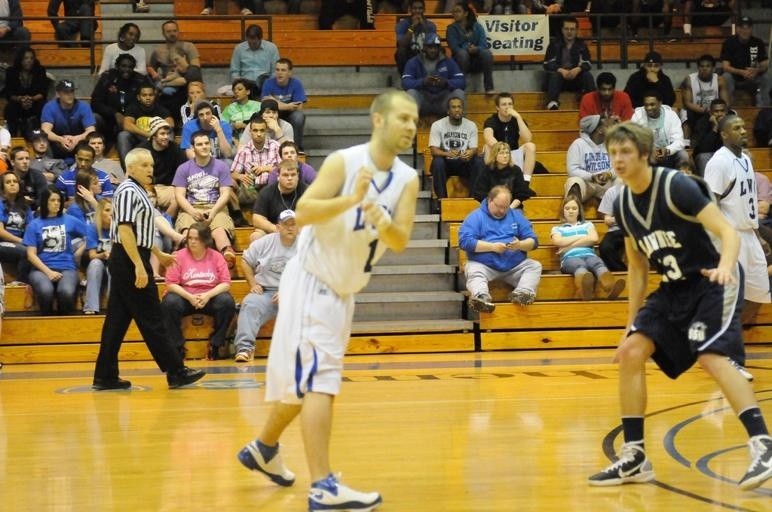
[506,241,513,246]
[433,75,440,81]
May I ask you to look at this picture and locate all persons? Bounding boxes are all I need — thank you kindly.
[589,121,772,489]
[237,88,422,512]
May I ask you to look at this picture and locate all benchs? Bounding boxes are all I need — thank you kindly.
[1,89,304,366]
[413,87,772,351]
[0,0,746,70]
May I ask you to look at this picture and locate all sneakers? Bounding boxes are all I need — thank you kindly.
[507,291,535,305]
[237,439,295,486]
[234,349,254,361]
[221,246,237,280]
[167,366,207,388]
[468,295,495,313]
[92,377,130,390]
[725,356,753,382]
[308,472,382,512]
[208,344,219,360]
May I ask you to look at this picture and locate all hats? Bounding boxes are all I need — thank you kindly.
[737,15,752,26]
[149,116,172,137]
[643,52,662,63]
[277,209,295,223]
[258,99,278,114]
[423,32,441,47]
[28,128,48,141]
[56,79,79,91]
[580,115,601,135]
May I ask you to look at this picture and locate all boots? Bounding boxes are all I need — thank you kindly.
[598,271,625,300]
[738,434,772,491]
[588,439,655,486]
[574,272,594,300]
